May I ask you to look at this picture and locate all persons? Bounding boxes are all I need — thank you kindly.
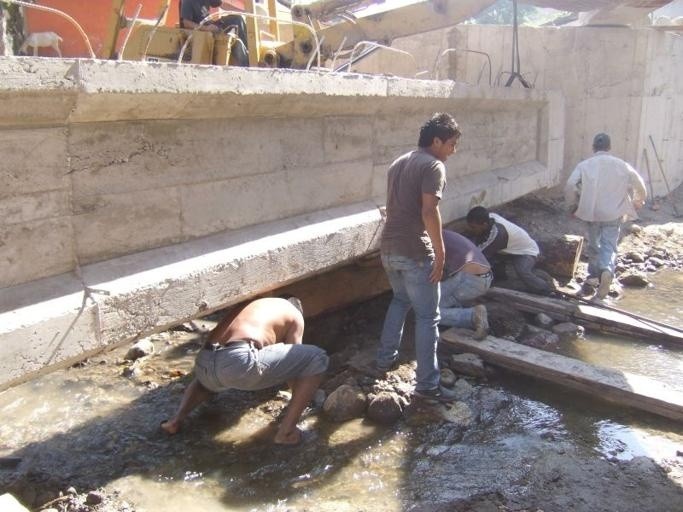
[562,132,647,300]
[157,294,332,448]
[376,110,463,403]
[177,1,249,67]
[460,205,551,295]
[439,228,495,342]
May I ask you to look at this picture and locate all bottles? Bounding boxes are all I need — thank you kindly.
[594,133,610,148]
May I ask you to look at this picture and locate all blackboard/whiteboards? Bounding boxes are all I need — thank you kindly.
[101,1,671,69]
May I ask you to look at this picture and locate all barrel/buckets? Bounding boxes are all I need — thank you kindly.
[271,431,318,455]
[157,418,176,436]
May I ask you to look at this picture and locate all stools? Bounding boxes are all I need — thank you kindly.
[475,271,491,278]
[204,341,246,351]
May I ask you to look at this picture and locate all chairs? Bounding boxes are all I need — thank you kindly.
[472,305,488,339]
[414,383,457,401]
[377,352,407,370]
[587,269,612,299]
[487,313,505,337]
